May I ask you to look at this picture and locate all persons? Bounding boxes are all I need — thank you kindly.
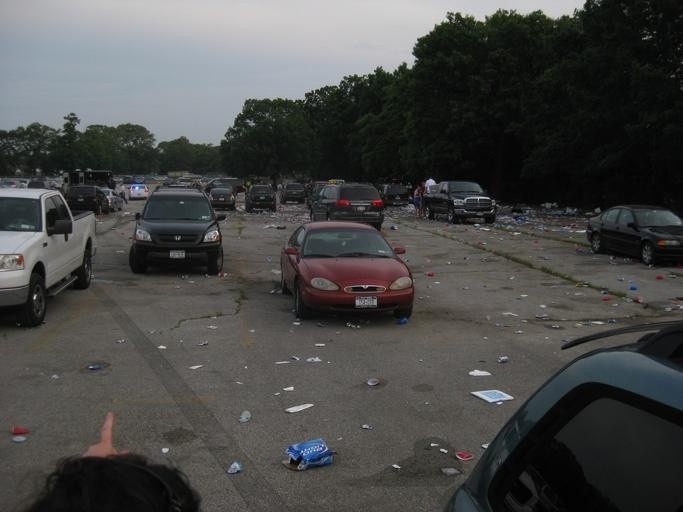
[414,175,436,218]
[15,410,201,512]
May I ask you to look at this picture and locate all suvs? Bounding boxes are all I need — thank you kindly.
[128,185,227,276]
[309,182,383,229]
[376,178,410,206]
[441,318,682,511]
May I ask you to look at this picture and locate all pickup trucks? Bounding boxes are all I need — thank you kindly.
[422,181,497,226]
[0,187,96,326]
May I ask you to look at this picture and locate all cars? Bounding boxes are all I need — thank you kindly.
[585,203,683,267]
[279,221,415,321]
